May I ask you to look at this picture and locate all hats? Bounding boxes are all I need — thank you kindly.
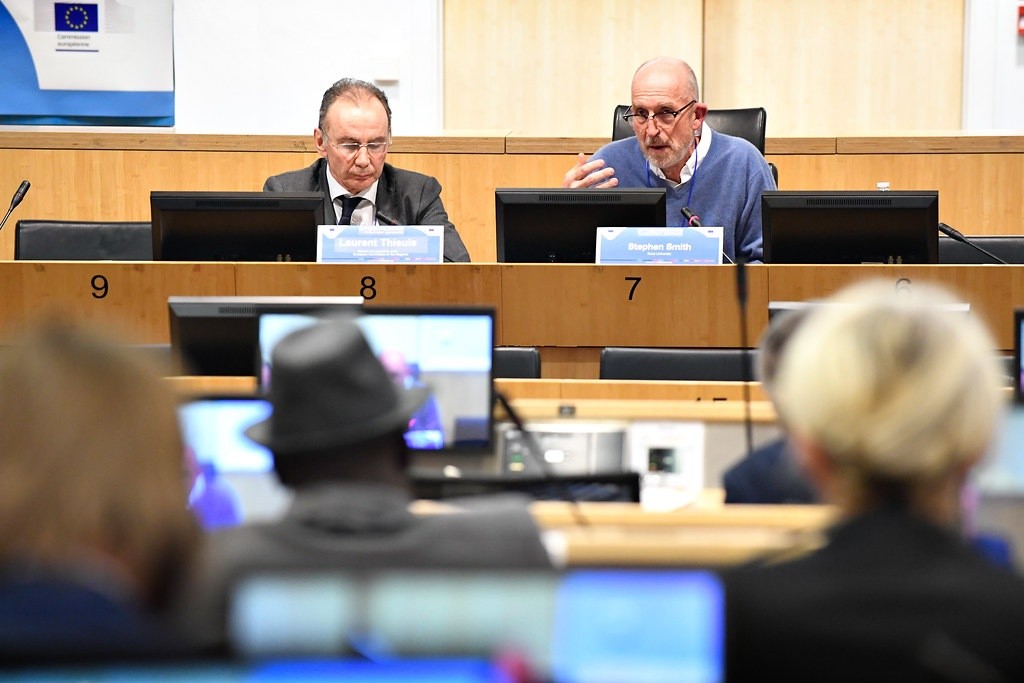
[242,319,429,449]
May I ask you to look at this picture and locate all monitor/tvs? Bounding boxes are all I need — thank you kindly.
[768,300,969,384]
[1,295,743,683]
[1013,307,1024,405]
[495,188,666,264]
[760,190,939,265]
[149,191,325,262]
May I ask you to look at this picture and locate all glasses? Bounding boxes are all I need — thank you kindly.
[322,130,395,156]
[623,99,696,127]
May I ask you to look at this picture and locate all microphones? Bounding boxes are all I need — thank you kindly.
[681,206,734,264]
[494,392,581,514]
[375,210,456,264]
[937,223,1007,264]
[734,256,754,458]
[0,180,29,230]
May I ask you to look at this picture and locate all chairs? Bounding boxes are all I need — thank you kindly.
[614,106,774,190]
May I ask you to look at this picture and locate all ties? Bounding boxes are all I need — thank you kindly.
[336,195,367,225]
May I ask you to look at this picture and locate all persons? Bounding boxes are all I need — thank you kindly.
[0,307,202,650]
[721,280,1024,683]
[199,323,558,646]
[722,300,825,503]
[380,350,440,431]
[563,57,778,264]
[262,79,471,263]
